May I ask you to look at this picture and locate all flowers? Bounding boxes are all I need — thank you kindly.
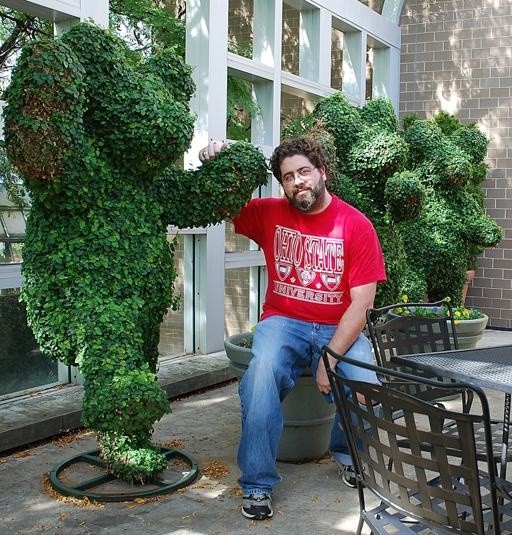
[394,294,484,327]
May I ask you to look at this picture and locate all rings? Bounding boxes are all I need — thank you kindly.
[203,151,208,157]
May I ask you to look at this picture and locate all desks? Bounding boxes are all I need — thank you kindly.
[385,341,511,495]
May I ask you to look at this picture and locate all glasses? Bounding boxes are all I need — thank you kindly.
[279,167,316,185]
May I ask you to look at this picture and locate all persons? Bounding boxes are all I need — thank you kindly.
[197,137,388,521]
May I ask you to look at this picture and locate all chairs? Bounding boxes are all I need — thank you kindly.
[321,342,510,535]
[359,303,512,493]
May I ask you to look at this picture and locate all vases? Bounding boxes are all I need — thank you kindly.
[225,331,338,464]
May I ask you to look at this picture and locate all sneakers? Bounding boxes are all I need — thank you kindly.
[242,493,273,520]
[334,459,365,488]
